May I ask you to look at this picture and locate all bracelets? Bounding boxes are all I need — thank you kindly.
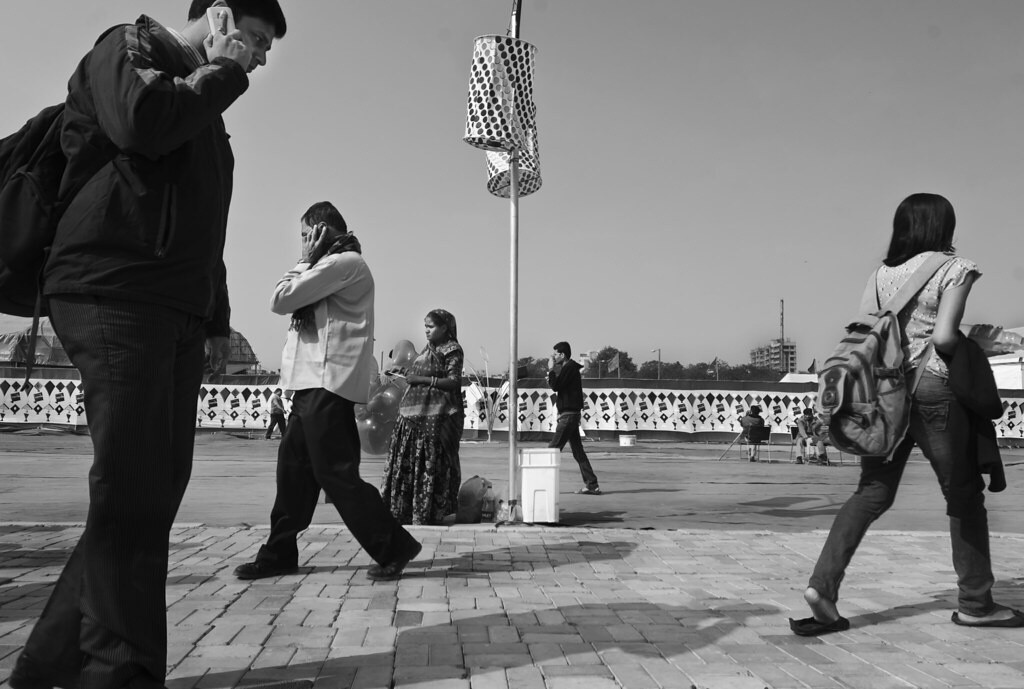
[430,376,438,387]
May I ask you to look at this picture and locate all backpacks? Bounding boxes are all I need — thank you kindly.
[811,250,957,461]
[0,103,120,317]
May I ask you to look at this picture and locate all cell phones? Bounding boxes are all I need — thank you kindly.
[206,7,236,38]
[316,222,327,239]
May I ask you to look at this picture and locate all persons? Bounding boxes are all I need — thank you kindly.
[232,201,421,580]
[378,309,463,524]
[544,341,600,494]
[787,192,1024,636]
[0,0,287,689]
[740,406,764,462]
[265,388,286,439]
[796,408,829,465]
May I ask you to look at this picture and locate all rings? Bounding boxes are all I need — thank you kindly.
[218,27,225,35]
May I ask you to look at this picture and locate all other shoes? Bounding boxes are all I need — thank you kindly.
[795,457,803,464]
[818,458,829,464]
[746,448,751,455]
[750,456,757,462]
[264,436,272,440]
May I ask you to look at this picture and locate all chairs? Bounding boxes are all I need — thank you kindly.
[738,423,772,463]
[790,426,843,464]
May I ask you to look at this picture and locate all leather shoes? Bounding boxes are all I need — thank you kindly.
[366,543,422,580]
[234,561,299,578]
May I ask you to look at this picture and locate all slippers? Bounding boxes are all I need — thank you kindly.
[951,602,1024,627]
[789,617,850,636]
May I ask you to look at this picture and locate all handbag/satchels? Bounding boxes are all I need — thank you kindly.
[455,474,493,524]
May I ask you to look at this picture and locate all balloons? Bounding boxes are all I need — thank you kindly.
[354,338,420,455]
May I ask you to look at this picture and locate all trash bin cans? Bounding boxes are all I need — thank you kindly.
[518,445,562,526]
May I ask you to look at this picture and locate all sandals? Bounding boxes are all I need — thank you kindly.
[574,487,601,495]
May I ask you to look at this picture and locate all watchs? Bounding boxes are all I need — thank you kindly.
[298,258,308,263]
